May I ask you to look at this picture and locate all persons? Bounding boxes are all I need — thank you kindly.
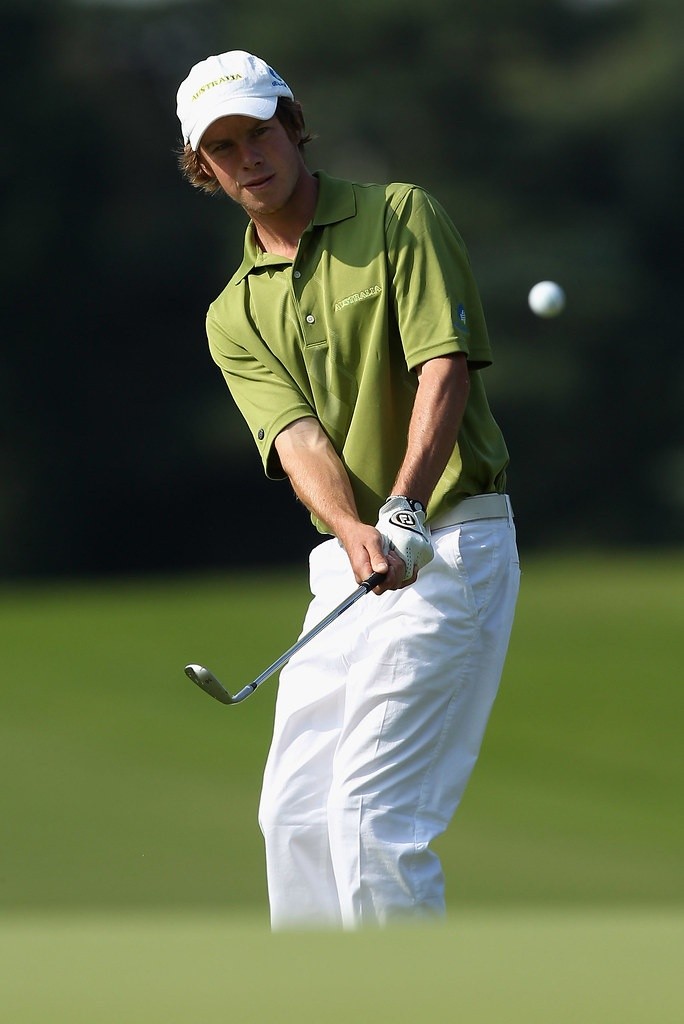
[176,50,520,933]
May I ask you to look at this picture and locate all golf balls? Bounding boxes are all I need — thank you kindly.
[527,279,567,321]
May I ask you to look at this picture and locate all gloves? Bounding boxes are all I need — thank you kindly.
[374,495,434,583]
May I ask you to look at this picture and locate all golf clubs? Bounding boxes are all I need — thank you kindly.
[183,571,388,707]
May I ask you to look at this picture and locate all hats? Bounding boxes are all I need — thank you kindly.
[175,50,294,151]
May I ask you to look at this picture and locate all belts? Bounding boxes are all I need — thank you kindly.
[425,495,515,531]
